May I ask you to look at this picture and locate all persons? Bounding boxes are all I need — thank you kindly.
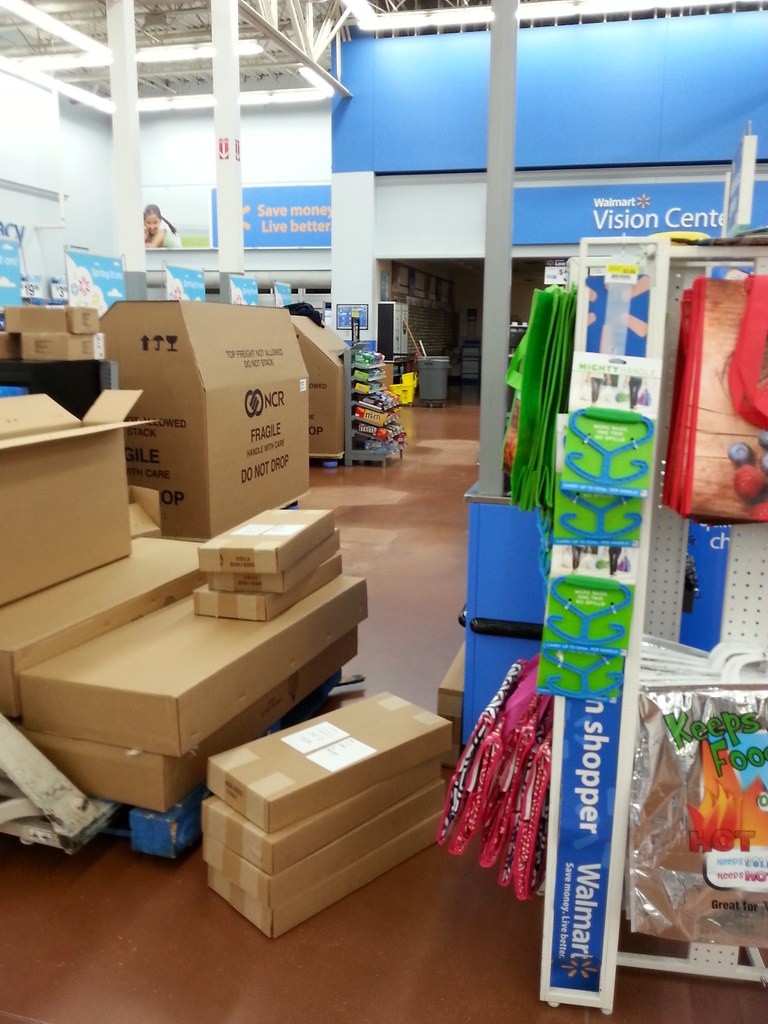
[142,203,181,249]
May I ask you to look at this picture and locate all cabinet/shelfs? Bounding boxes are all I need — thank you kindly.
[537,235,768,1018]
[344,348,403,468]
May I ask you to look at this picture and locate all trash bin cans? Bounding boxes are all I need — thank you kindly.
[415,355,451,408]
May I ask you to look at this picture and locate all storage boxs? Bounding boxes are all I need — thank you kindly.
[69,334,106,361]
[0,540,208,717]
[21,332,69,362]
[65,306,101,334]
[207,810,444,938]
[3,306,68,333]
[290,316,350,455]
[435,641,465,768]
[205,690,454,832]
[100,299,312,540]
[207,527,340,594]
[12,625,358,811]
[0,331,19,360]
[21,575,370,758]
[200,752,444,875]
[0,388,161,609]
[193,552,343,621]
[198,509,337,573]
[128,483,162,537]
[203,779,446,909]
